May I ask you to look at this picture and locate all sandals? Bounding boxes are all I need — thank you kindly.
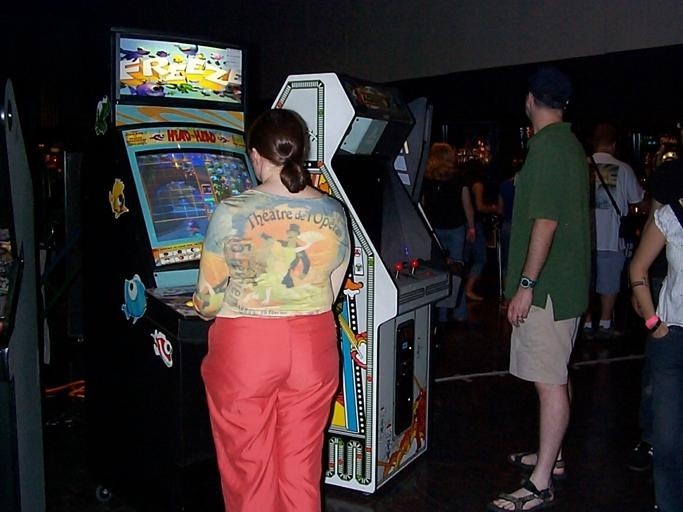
[508,449,569,483]
[486,482,557,512]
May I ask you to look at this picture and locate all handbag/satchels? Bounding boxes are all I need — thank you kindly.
[618,215,640,239]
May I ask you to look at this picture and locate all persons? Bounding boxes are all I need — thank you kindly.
[192,107,353,511]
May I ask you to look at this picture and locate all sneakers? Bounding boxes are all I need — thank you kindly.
[582,323,626,345]
[439,289,485,335]
[629,437,654,472]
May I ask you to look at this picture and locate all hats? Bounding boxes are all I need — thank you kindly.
[528,66,572,109]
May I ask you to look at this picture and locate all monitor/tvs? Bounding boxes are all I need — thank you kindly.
[114,121,262,271]
[336,164,450,263]
